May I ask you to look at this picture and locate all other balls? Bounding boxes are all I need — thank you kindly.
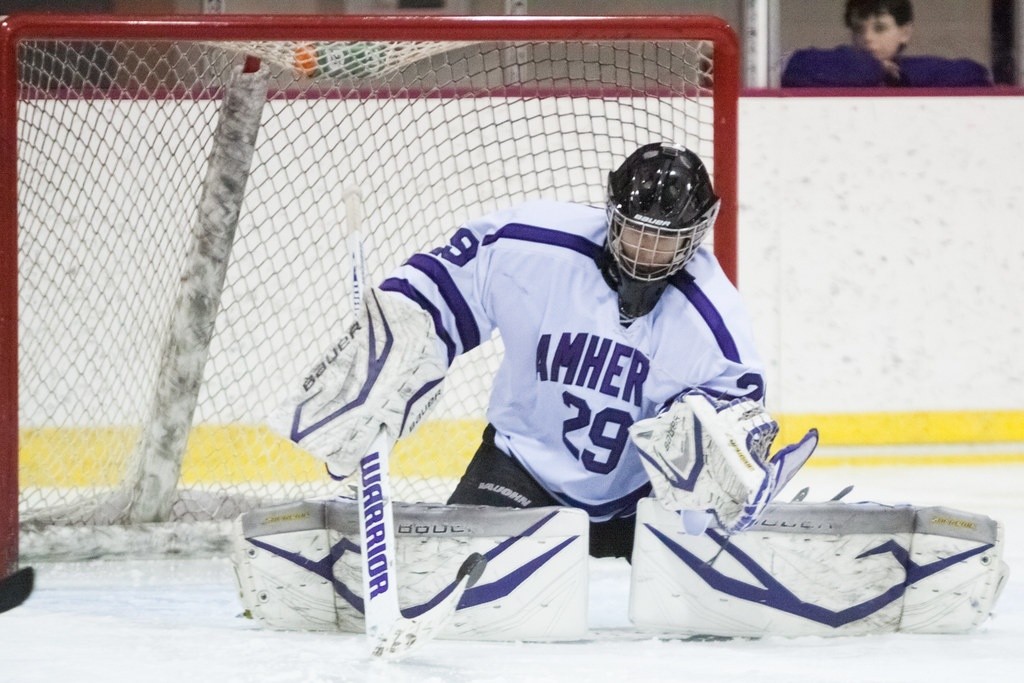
[455,551,489,590]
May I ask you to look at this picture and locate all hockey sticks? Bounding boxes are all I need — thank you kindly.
[340,182,471,664]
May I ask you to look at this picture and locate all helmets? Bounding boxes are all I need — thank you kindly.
[609,143,719,236]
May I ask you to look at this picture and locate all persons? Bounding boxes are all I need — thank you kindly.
[291,142,819,564]
[780,1,996,88]
